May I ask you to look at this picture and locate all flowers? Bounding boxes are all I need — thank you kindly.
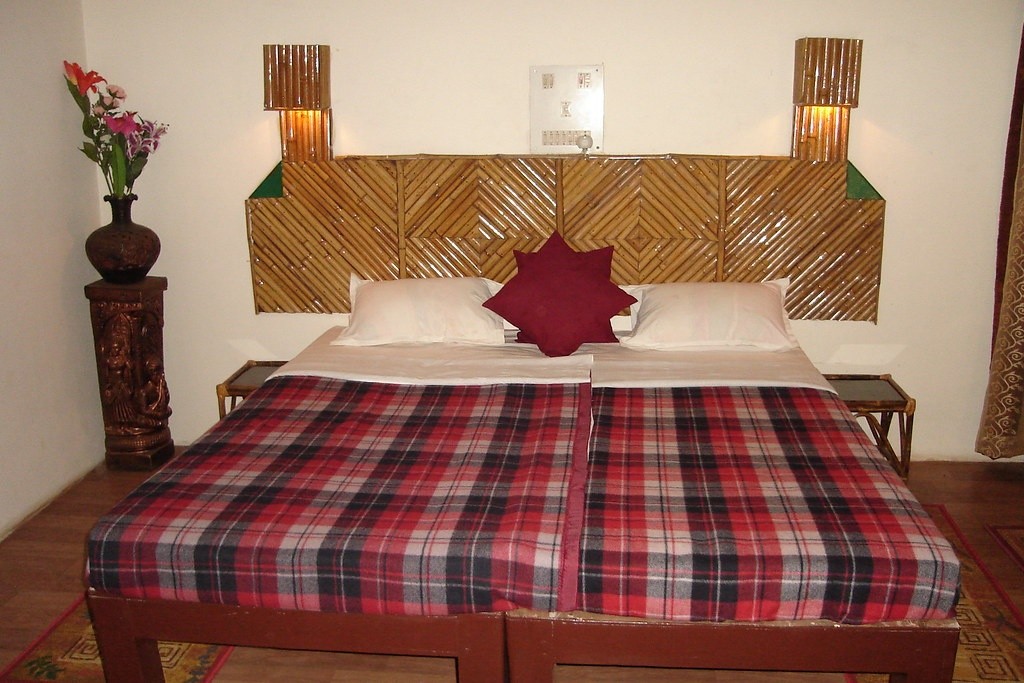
[62,59,172,197]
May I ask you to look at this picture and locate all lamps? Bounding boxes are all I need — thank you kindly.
[791,37,864,160]
[262,44,334,161]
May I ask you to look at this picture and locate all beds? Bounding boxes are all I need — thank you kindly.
[80,271,963,683]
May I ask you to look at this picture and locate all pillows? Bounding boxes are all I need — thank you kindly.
[615,277,800,352]
[482,231,638,358]
[328,272,506,348]
[513,245,620,343]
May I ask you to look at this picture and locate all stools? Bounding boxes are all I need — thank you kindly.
[822,373,916,486]
[216,360,288,420]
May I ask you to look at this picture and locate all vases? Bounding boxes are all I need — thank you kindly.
[85,194,161,284]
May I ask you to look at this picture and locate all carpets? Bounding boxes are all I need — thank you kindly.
[0,593,234,683]
[845,505,1024,683]
